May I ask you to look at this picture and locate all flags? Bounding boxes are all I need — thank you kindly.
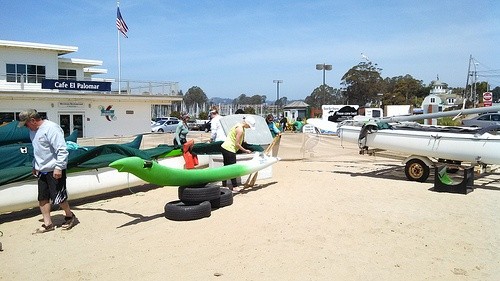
[116,7,130,37]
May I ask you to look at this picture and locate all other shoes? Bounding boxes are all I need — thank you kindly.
[35,223,56,233]
[62,213,80,230]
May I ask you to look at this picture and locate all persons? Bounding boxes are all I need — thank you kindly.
[208,107,226,141]
[17,108,77,233]
[265,114,305,138]
[220,115,256,191]
[174,115,190,145]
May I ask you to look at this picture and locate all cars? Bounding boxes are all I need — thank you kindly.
[461,113,500,131]
[200,121,211,133]
[151,117,179,132]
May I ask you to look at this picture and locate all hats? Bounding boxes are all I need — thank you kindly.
[17,109,38,127]
[244,115,256,130]
